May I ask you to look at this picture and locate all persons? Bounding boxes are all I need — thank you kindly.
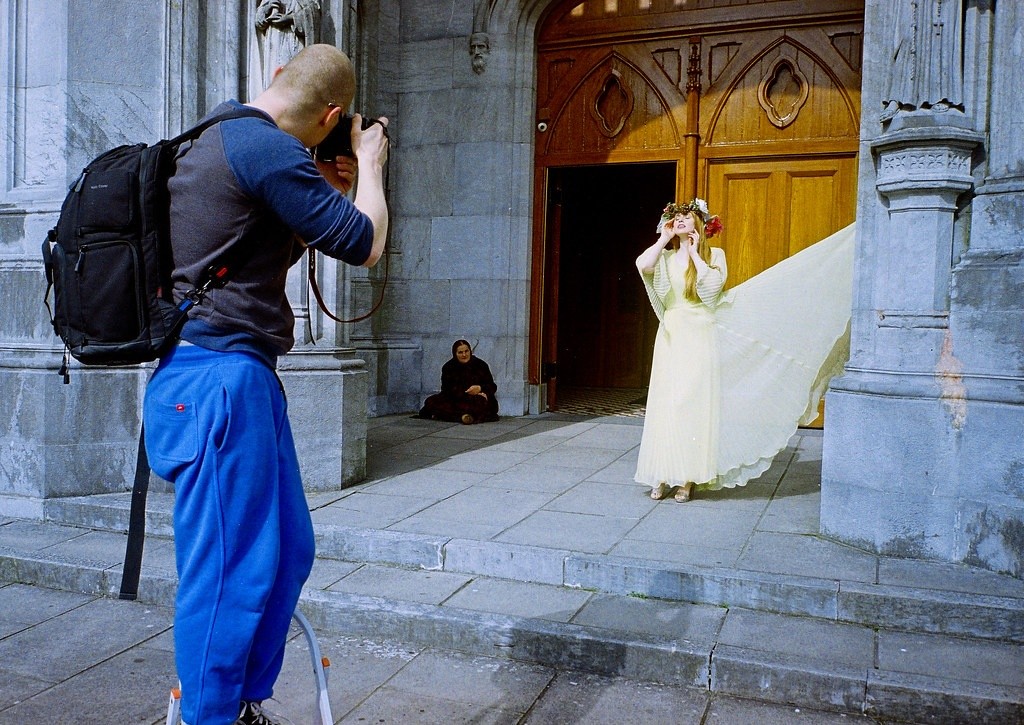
[419,339,499,424]
[635,197,728,503]
[142,43,391,725]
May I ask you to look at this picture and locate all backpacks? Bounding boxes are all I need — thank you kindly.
[41,108,277,384]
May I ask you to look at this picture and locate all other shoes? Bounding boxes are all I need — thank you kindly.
[462,414,473,424]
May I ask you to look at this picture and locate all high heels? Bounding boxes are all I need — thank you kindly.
[675,483,695,503]
[651,483,665,499]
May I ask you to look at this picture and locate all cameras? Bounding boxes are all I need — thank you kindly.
[310,113,376,163]
